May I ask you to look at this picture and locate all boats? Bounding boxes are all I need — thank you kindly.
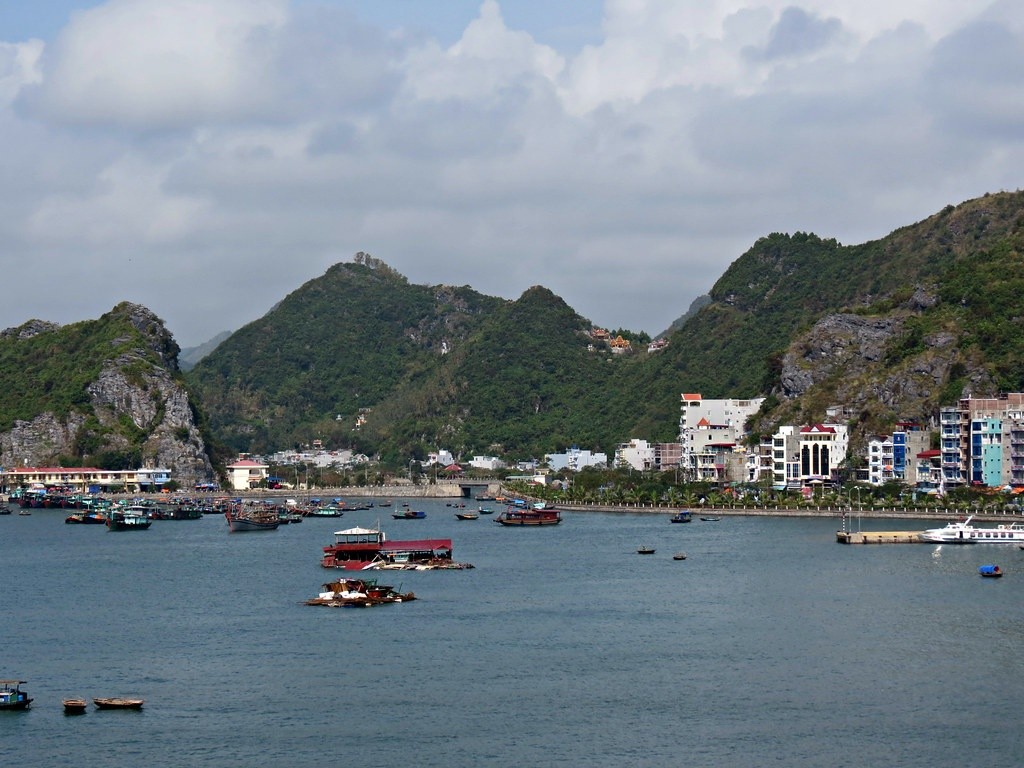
[0,679,34,710]
[92,697,144,709]
[699,517,720,522]
[637,545,657,554]
[454,509,480,520]
[669,511,694,523]
[320,525,475,571]
[492,504,563,526]
[61,695,87,710]
[916,515,1024,543]
[0,484,556,531]
[391,507,428,519]
[297,577,417,607]
[672,555,688,560]
[105,511,153,531]
[978,565,1003,577]
